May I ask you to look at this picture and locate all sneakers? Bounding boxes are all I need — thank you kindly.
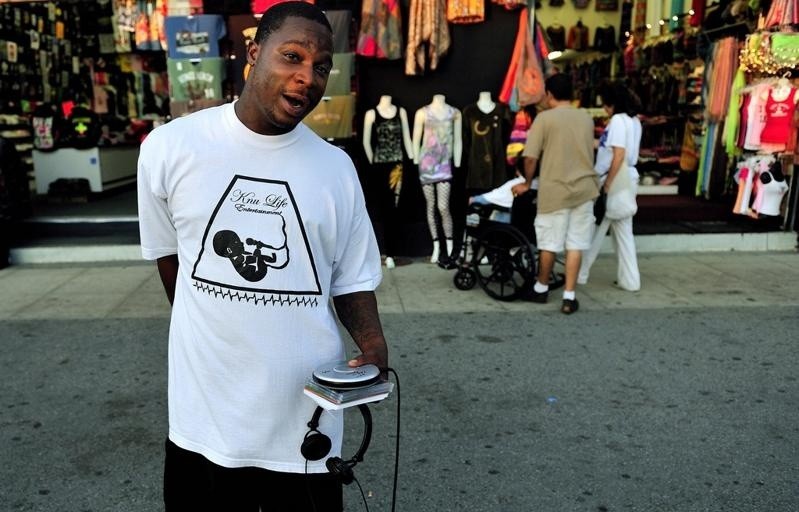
[520,282,580,314]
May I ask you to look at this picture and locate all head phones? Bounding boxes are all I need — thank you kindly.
[301,404,372,485]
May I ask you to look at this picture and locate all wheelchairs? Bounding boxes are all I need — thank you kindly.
[454,187,564,302]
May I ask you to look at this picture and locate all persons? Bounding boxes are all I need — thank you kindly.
[752,161,789,216]
[576,83,641,291]
[761,79,799,143]
[457,150,539,266]
[513,73,601,313]
[137,2,386,512]
[363,92,509,269]
